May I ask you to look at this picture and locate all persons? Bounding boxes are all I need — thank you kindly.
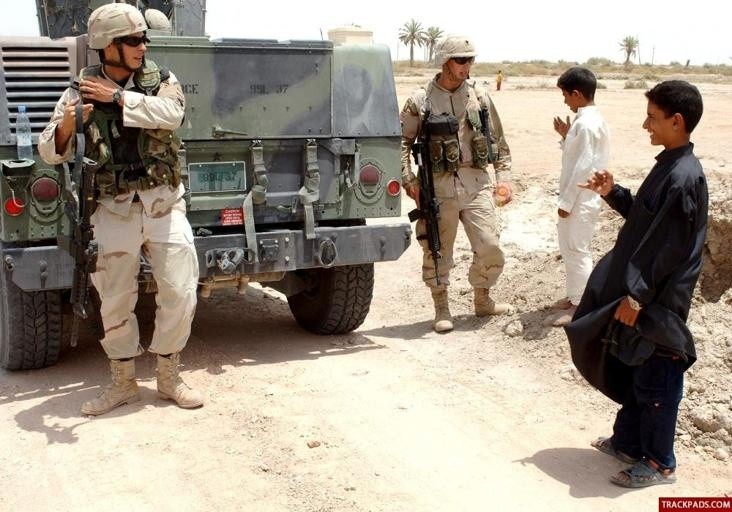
[399,35,514,332]
[38,3,205,415]
[575,79,708,488]
[495,70,502,91]
[550,66,611,327]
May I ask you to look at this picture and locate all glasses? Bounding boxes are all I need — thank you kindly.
[116,33,147,48]
[450,57,476,66]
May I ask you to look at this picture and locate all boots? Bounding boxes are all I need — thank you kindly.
[81,358,146,415]
[431,290,454,332]
[156,351,204,411]
[473,286,513,317]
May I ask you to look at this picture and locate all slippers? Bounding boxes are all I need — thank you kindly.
[593,436,645,464]
[610,460,676,488]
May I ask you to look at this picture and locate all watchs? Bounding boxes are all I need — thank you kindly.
[111,88,124,104]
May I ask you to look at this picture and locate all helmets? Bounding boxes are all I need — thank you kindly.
[84,4,149,74]
[433,33,479,82]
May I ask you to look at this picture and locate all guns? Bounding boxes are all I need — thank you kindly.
[407,137,443,287]
[50,154,98,347]
